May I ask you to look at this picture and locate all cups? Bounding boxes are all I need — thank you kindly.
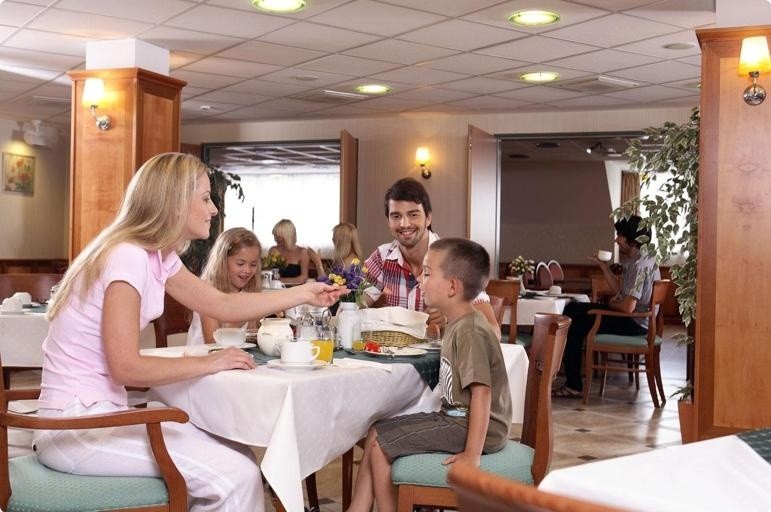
[1,298,23,311]
[270,280,285,289]
[213,328,246,346]
[549,286,561,294]
[597,250,612,261]
[11,292,31,305]
[311,331,334,363]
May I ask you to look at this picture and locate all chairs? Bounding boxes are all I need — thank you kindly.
[0,274,63,389]
[536,259,564,290]
[447,452,639,512]
[486,281,520,344]
[396,312,572,512]
[583,279,671,408]
[0,348,189,512]
[153,292,193,348]
[590,273,620,303]
[660,280,679,315]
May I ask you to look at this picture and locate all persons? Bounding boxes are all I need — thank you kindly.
[358,178,501,344]
[346,238,512,512]
[32,151,351,512]
[187,228,261,343]
[551,215,661,398]
[307,223,368,289]
[268,220,309,285]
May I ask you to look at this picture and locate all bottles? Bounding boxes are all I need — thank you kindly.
[338,302,362,351]
[300,312,315,339]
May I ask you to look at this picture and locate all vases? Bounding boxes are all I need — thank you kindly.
[507,275,525,291]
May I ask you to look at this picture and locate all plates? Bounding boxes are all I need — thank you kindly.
[0,309,31,314]
[203,342,441,370]
[284,282,301,286]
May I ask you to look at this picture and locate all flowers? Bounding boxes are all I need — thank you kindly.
[316,258,369,289]
[270,248,288,270]
[509,256,536,275]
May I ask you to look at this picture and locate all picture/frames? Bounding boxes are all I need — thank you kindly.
[2,151,35,196]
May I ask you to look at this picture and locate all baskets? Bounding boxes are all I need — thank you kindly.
[361,331,429,346]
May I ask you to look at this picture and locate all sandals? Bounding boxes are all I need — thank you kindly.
[551,386,584,399]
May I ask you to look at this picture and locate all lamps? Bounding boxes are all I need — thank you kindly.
[738,36,770,107]
[81,78,111,131]
[415,147,431,179]
